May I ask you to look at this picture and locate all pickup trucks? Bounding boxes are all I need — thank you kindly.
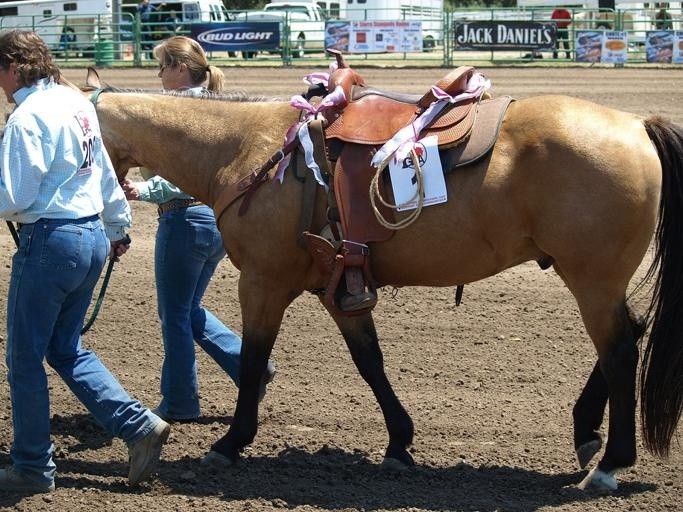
[235,2,327,60]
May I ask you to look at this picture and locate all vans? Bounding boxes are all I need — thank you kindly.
[116,0,231,64]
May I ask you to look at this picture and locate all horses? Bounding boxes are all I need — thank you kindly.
[53,64,683,497]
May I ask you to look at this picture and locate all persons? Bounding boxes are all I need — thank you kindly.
[548,4,570,59]
[120,35,278,424]
[651,2,673,31]
[0,28,172,494]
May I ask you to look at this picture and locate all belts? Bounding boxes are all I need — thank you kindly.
[159,199,203,214]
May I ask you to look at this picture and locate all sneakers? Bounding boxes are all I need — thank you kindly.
[149,407,198,423]
[259,366,275,404]
[0,465,56,493]
[127,417,170,483]
